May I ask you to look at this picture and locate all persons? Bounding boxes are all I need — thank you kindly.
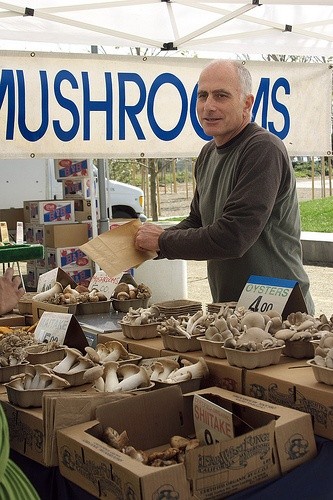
[133,58,317,322]
[0,268,25,315]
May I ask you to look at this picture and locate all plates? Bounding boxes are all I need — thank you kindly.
[149,299,202,322]
[206,301,238,316]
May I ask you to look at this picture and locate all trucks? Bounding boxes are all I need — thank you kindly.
[0,158,145,235]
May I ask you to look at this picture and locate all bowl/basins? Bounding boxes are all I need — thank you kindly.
[17,301,33,315]
[65,302,77,314]
[115,297,150,312]
[306,359,333,385]
[119,321,162,340]
[43,360,95,386]
[78,300,112,315]
[0,360,30,384]
[160,333,204,353]
[282,339,314,359]
[221,344,286,369]
[85,352,142,376]
[196,336,227,359]
[3,383,65,408]
[24,344,67,364]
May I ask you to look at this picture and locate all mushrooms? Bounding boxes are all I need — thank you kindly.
[31,282,152,305]
[122,306,333,371]
[100,426,206,467]
[0,327,208,390]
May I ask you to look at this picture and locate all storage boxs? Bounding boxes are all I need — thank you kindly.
[0,157,333,499]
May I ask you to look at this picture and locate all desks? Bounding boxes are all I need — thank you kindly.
[0,240,44,294]
